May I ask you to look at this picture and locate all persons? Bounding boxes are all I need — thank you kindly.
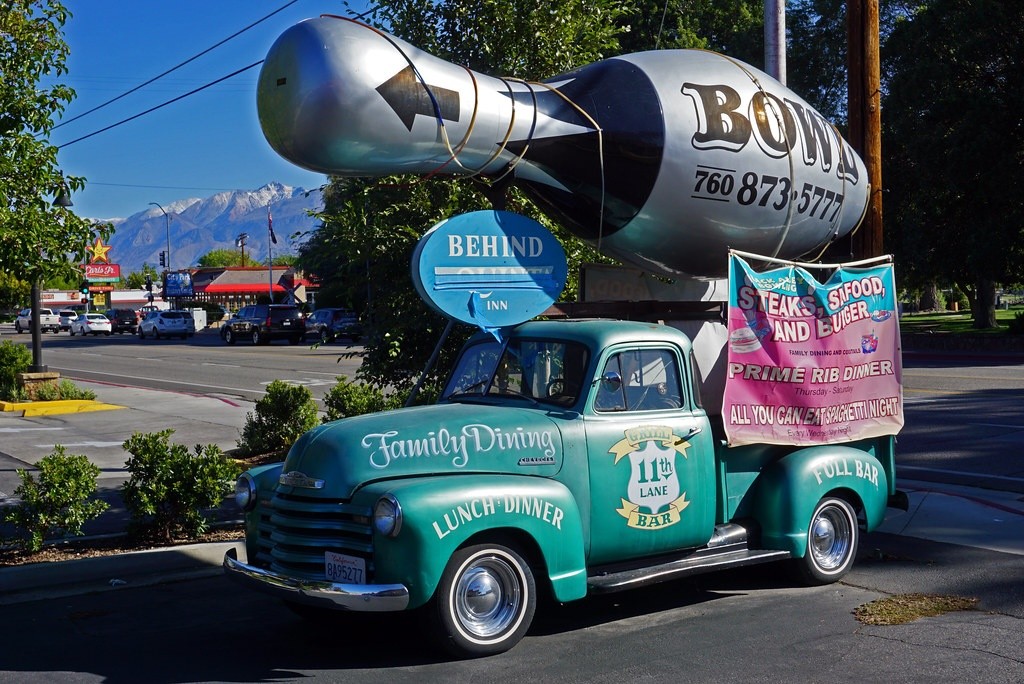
[557,356,624,410]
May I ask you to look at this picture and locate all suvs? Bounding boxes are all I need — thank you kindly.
[104,308,138,334]
[178,310,195,338]
[221,303,306,346]
[306,308,362,344]
[138,310,188,340]
[53,309,78,332]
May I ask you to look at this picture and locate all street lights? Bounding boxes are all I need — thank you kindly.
[148,202,171,267]
[27,170,73,374]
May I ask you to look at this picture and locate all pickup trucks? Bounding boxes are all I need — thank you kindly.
[222,319,911,654]
[15,308,61,334]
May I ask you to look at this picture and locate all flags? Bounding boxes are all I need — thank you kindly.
[268,212,278,245]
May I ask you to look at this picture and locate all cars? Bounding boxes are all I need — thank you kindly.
[69,313,112,337]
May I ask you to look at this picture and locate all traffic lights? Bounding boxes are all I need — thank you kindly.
[159,251,166,268]
[80,280,89,304]
[145,276,152,291]
